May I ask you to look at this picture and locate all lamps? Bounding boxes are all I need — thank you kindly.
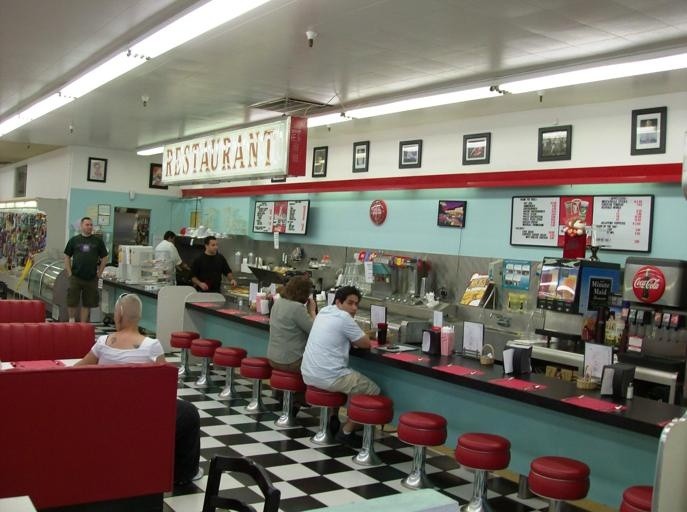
[537,91,543,103]
[0,1,265,137]
[134,46,686,157]
[68,125,75,135]
[140,95,149,107]
[306,30,318,49]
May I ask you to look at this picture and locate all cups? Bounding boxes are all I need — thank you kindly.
[377,322,387,344]
[564,198,589,222]
[440,330,455,357]
[255,293,265,312]
[260,300,269,314]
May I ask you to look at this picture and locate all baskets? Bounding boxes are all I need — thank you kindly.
[577,365,597,389]
[480,344,494,364]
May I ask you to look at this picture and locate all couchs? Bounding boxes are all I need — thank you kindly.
[0,299,45,323]
[0,364,178,510]
[0,322,95,362]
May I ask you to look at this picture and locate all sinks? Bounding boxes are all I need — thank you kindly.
[439,303,485,324]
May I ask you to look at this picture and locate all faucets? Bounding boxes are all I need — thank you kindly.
[488,311,504,322]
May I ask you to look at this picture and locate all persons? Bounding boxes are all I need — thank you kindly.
[299,284,380,445]
[189,235,237,293]
[71,293,200,492]
[154,230,191,286]
[63,217,109,324]
[266,275,317,419]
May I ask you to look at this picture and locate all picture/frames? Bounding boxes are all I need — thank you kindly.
[98,203,112,216]
[461,131,491,167]
[252,198,311,237]
[86,157,107,182]
[148,163,169,190]
[398,139,424,169]
[369,303,387,334]
[508,192,656,257]
[351,141,370,173]
[311,146,328,177]
[437,200,467,229]
[271,176,286,183]
[582,340,614,387]
[97,215,110,226]
[628,104,668,157]
[461,320,485,359]
[248,280,260,307]
[536,124,572,162]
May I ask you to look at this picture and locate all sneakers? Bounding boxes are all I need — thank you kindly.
[335,429,362,448]
[329,414,340,436]
[296,399,312,408]
[178,467,204,484]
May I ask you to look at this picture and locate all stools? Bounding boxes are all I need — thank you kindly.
[453,431,511,511]
[528,455,589,510]
[240,356,272,412]
[271,370,303,427]
[619,484,651,511]
[394,410,447,491]
[302,385,346,445]
[348,394,393,465]
[214,346,247,401]
[171,331,199,379]
[189,339,221,388]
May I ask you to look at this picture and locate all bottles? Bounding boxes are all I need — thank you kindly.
[594,306,604,343]
[643,270,651,298]
[626,382,633,400]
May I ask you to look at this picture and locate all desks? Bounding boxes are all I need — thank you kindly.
[0,358,83,369]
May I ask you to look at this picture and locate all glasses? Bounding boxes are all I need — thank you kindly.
[118,293,127,320]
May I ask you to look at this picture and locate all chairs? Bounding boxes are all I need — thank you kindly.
[197,454,281,511]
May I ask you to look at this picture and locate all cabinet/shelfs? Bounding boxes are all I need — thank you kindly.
[27,257,90,323]
